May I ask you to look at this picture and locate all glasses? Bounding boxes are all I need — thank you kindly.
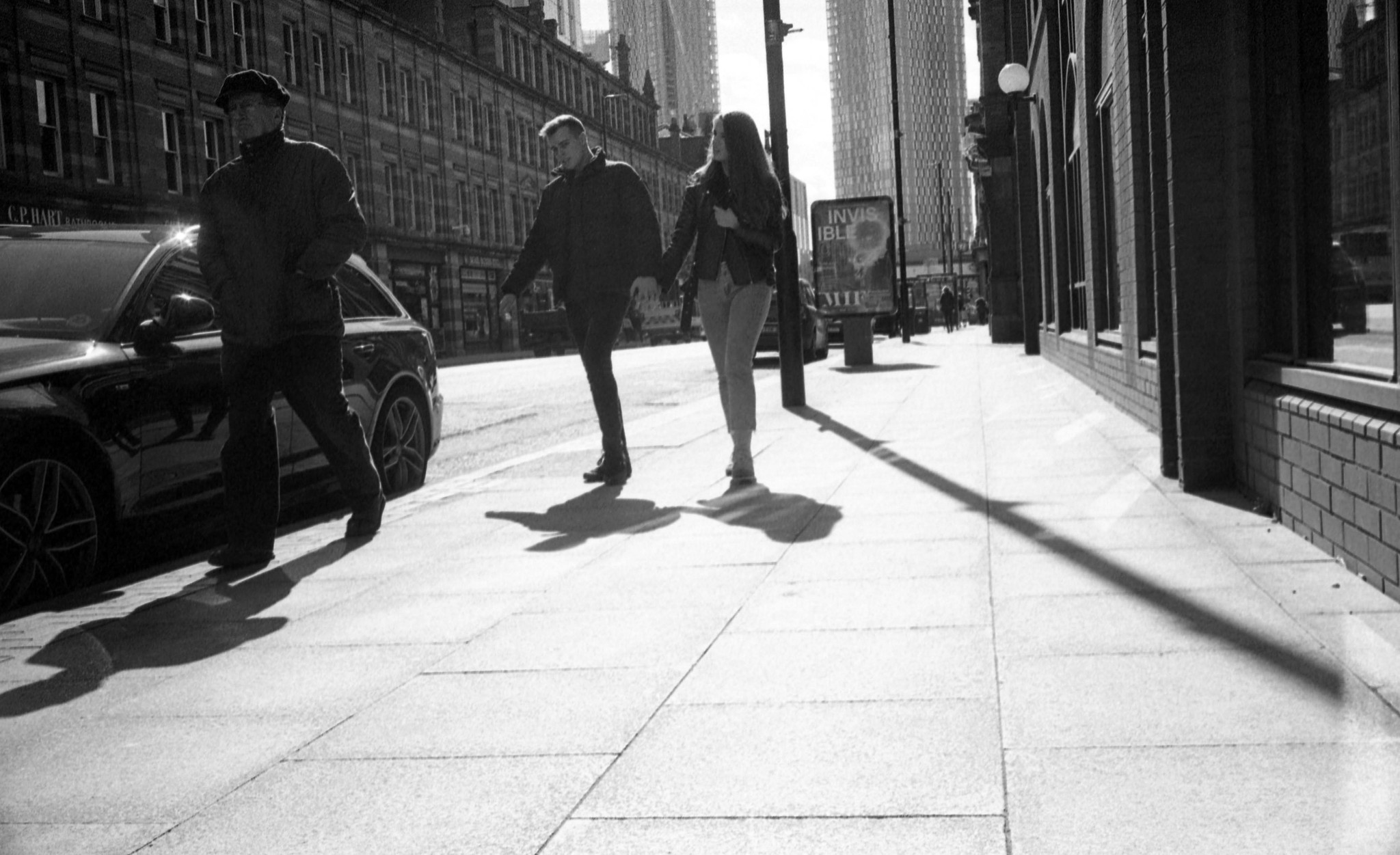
[228,101,276,111]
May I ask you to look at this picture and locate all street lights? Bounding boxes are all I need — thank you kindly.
[997,63,1041,354]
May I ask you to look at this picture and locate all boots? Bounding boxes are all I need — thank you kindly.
[725,429,756,482]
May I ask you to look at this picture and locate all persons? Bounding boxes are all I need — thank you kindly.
[637,112,785,481]
[497,114,660,482]
[197,69,385,567]
[938,286,989,333]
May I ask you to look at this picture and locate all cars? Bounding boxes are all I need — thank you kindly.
[0,222,447,612]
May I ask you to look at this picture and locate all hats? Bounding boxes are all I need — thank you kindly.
[215,69,290,109]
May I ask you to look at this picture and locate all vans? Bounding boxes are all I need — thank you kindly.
[751,277,829,362]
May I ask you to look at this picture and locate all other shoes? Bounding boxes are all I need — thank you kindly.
[583,452,633,484]
[345,495,387,540]
[207,541,274,567]
